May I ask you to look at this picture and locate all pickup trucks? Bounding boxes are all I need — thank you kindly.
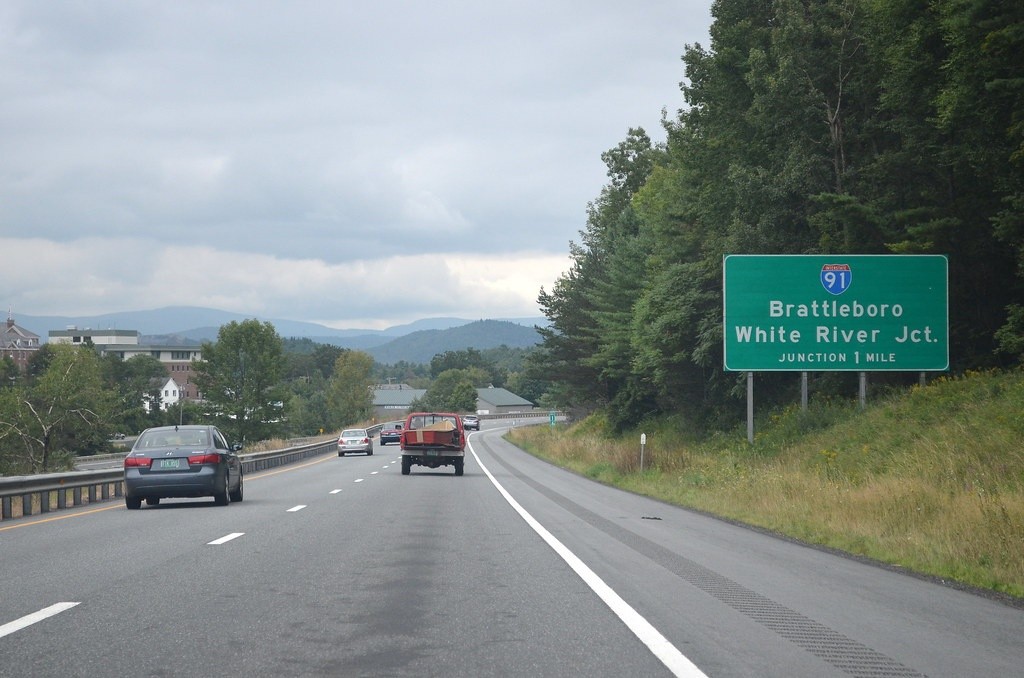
[400,411,466,476]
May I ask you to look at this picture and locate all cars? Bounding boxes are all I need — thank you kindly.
[379,422,405,445]
[462,415,481,431]
[123,424,244,509]
[337,429,374,457]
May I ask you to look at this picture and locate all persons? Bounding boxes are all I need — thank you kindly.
[414,418,423,429]
